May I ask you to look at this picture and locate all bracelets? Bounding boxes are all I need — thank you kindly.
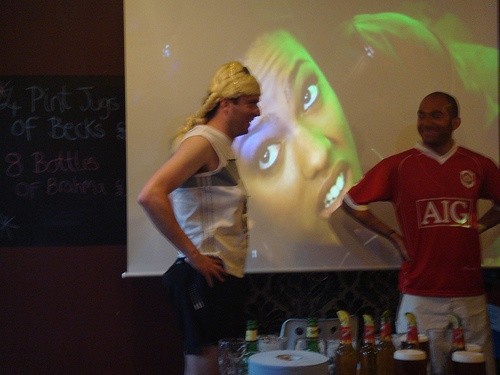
[384,229,396,238]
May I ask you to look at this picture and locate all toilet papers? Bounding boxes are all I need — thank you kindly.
[247,349,329,375]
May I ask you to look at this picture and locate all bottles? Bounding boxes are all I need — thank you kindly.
[403,312,419,350]
[303,318,320,353]
[240,321,259,375]
[332,310,357,375]
[360,309,397,375]
[446,310,466,375]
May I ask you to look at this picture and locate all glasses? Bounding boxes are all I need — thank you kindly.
[223,67,250,80]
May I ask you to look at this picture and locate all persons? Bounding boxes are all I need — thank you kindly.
[341,91,500,375]
[138,62,262,375]
[157,12,499,265]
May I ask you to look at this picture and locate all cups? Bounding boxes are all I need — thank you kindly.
[428,328,453,375]
[451,342,487,375]
[257,334,398,374]
[392,333,429,375]
[217,339,247,375]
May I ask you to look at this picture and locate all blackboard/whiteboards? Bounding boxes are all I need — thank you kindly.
[0,73,128,251]
